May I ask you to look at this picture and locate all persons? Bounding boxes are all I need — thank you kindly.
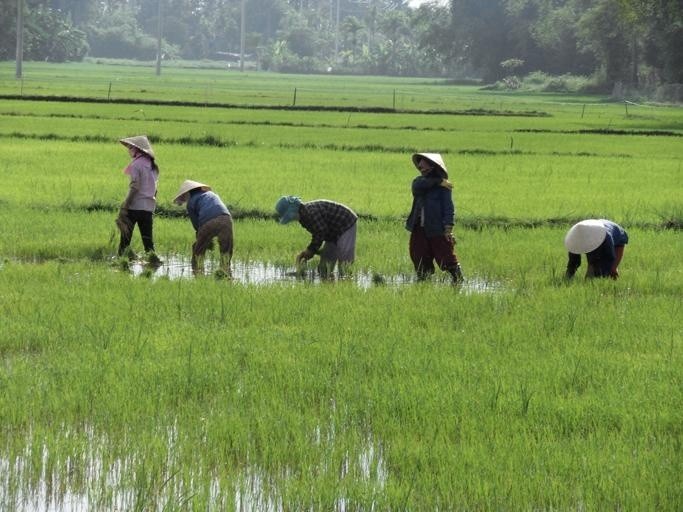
[118,135,158,256]
[274,196,358,277]
[405,151,464,283]
[564,219,628,279]
[174,179,233,269]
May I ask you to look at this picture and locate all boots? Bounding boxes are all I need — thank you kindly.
[446,263,464,282]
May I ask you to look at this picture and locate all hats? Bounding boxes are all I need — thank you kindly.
[171,180,211,205]
[564,220,607,255]
[411,152,448,181]
[118,134,154,160]
